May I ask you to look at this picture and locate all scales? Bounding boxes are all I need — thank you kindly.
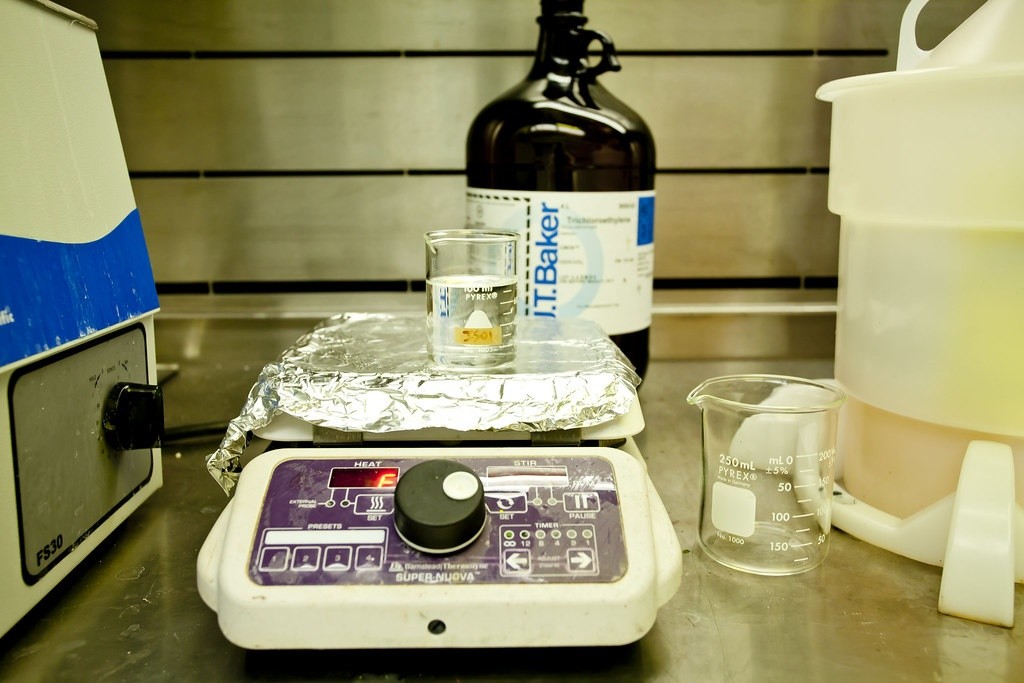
[195,310,686,651]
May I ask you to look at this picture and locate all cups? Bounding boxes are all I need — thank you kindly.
[423,228,521,372]
[687,374,846,577]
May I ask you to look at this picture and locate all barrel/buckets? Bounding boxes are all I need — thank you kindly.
[816,62,1023,521]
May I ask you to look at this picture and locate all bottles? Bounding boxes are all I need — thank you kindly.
[466,0,658,395]
